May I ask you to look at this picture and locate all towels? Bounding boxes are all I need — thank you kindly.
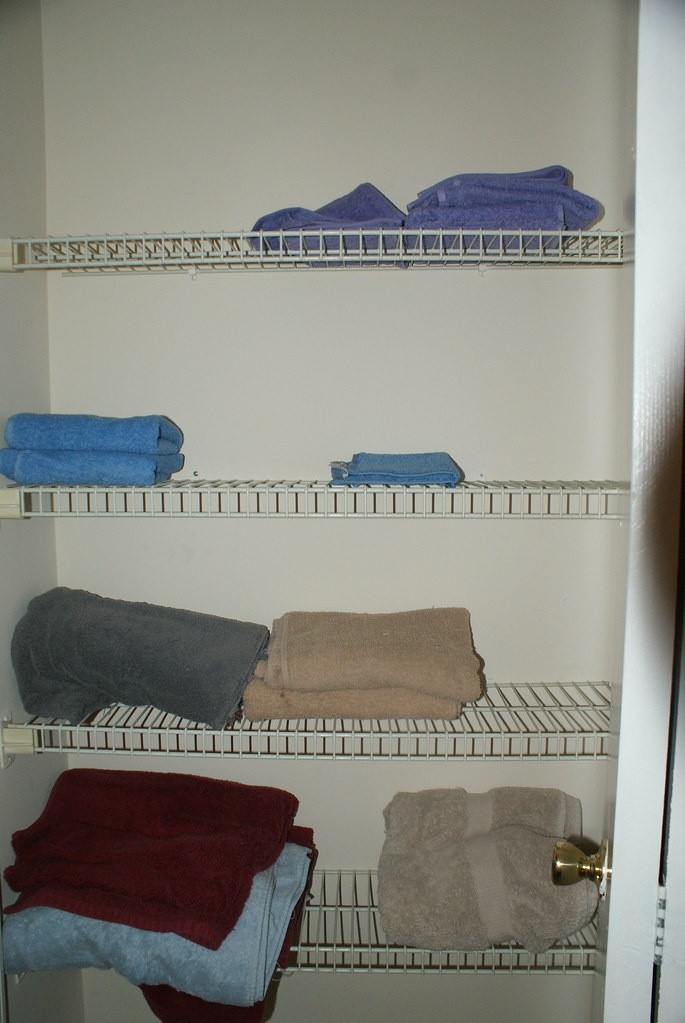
[374,783,601,959]
[0,768,324,1023]
[248,159,603,259]
[255,604,490,702]
[0,446,177,490]
[240,673,470,727]
[3,409,188,457]
[6,583,273,736]
[328,450,467,488]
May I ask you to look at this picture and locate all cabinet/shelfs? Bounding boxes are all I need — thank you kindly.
[0,0,685,1023]
